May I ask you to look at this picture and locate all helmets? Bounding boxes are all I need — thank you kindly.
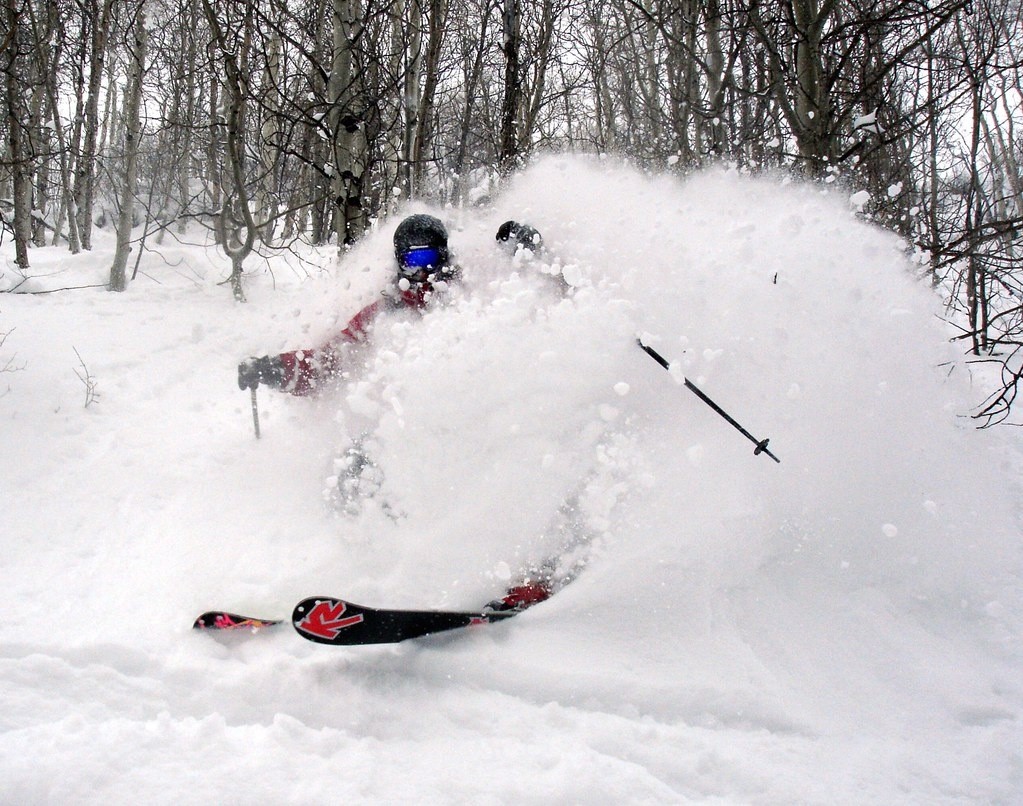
[393,213,463,288]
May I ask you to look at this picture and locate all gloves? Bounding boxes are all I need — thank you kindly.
[238,354,284,390]
[496,221,543,256]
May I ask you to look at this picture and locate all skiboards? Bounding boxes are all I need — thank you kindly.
[191,535,604,645]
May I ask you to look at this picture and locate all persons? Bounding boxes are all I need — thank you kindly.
[239,214,576,500]
